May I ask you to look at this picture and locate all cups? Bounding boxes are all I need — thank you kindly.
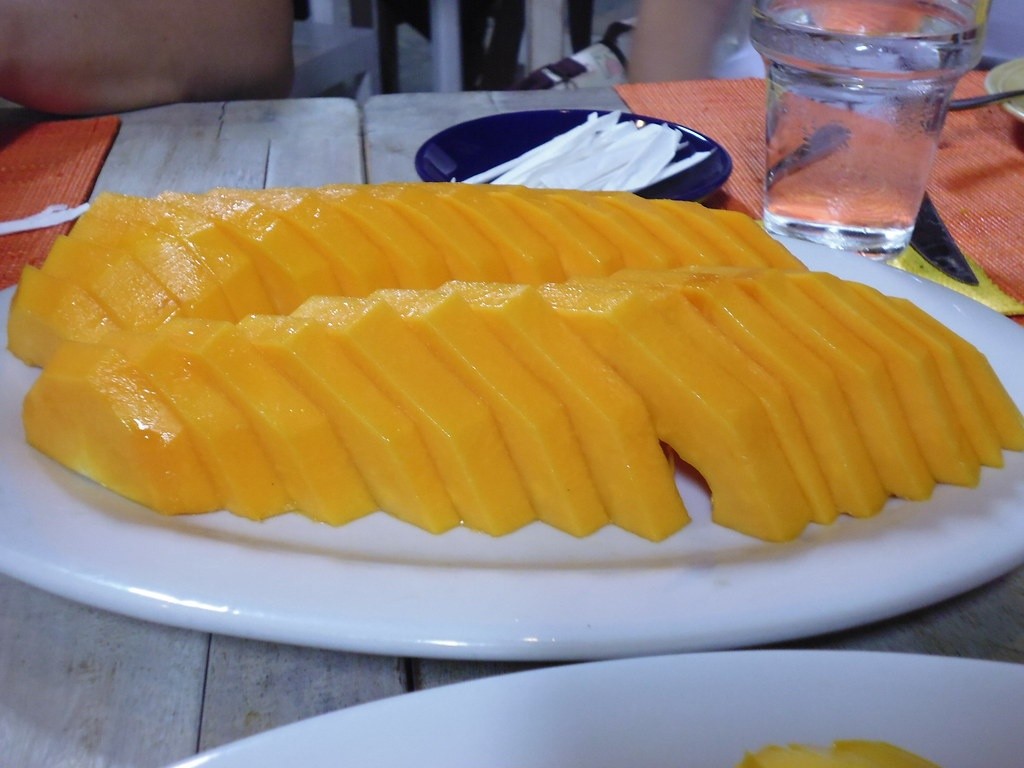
[749,0,988,260]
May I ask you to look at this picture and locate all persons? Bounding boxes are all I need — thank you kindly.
[523,0,1023,89]
[0,0,294,116]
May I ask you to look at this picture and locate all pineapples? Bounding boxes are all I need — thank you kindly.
[738,738,943,768]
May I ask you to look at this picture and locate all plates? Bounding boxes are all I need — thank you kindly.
[415,109,733,202]
[170,649,1024,768]
[0,236,1024,660]
[984,57,1024,122]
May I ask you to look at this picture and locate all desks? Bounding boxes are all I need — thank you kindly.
[0,88,1023,767]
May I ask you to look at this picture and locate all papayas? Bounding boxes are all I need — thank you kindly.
[8,181,1024,544]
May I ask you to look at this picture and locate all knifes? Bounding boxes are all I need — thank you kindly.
[908,191,980,286]
